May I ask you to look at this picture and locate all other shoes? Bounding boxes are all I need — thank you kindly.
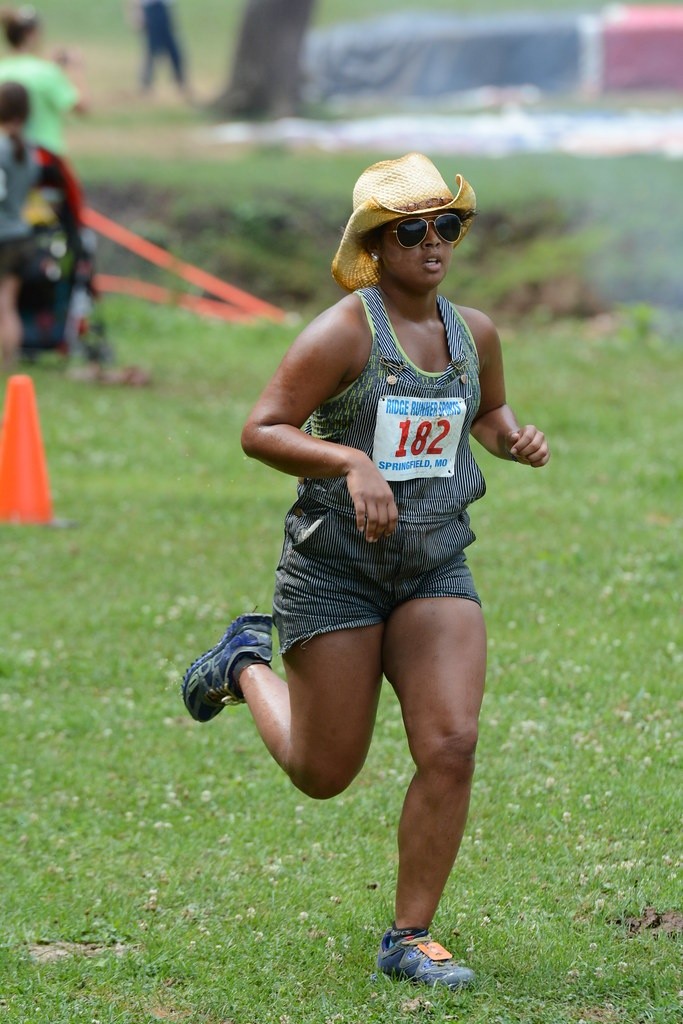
[22,340,70,366]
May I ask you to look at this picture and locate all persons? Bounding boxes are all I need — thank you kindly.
[0,8,148,388]
[139,0,190,95]
[181,152,550,993]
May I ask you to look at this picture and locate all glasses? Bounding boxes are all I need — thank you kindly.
[381,212,462,249]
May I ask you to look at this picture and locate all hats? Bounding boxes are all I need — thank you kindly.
[331,153,476,292]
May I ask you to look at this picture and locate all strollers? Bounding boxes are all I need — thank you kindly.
[19,145,101,365]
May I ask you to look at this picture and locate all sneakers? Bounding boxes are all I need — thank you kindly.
[182,613,272,723]
[377,927,477,991]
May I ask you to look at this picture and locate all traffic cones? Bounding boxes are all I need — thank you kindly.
[0,374,57,526]
